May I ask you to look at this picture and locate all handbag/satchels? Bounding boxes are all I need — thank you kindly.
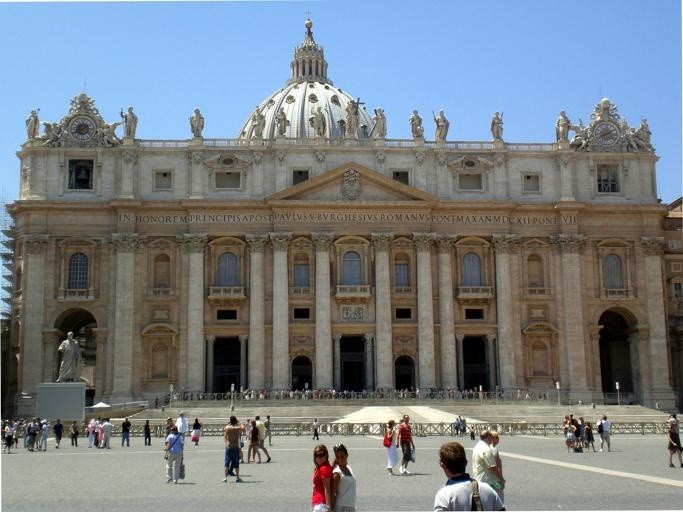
[383,432,392,448]
[597,424,604,433]
[471,479,484,511]
[179,464,185,479]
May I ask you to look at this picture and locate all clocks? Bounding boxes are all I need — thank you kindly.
[67,115,97,142]
[593,121,620,147]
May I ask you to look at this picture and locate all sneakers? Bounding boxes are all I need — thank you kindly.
[566,442,612,454]
[2,444,47,453]
[669,462,683,468]
[235,458,272,464]
[166,479,178,484]
[222,478,242,483]
[398,467,412,476]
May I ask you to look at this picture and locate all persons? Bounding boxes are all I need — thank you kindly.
[454,415,475,440]
[55,331,81,382]
[190,107,204,137]
[251,106,265,137]
[381,111,388,136]
[373,107,384,137]
[24,109,41,138]
[120,107,138,137]
[313,106,324,137]
[43,120,121,145]
[152,383,583,411]
[222,415,271,482]
[435,431,507,511]
[0,418,48,454]
[564,415,611,452]
[144,420,151,446]
[386,415,415,475]
[346,100,360,134]
[555,109,570,142]
[71,421,78,447]
[122,418,131,446]
[571,116,657,154]
[312,444,356,512]
[88,417,112,449]
[408,109,424,137]
[490,111,504,142]
[275,107,286,135]
[434,109,450,141]
[312,419,319,440]
[53,419,63,448]
[667,413,683,468]
[164,411,201,483]
